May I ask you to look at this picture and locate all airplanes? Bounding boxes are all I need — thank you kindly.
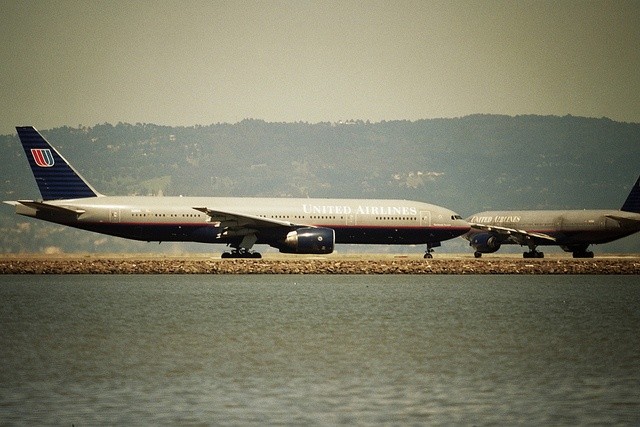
[461,177,640,258]
[4,128,470,260]
